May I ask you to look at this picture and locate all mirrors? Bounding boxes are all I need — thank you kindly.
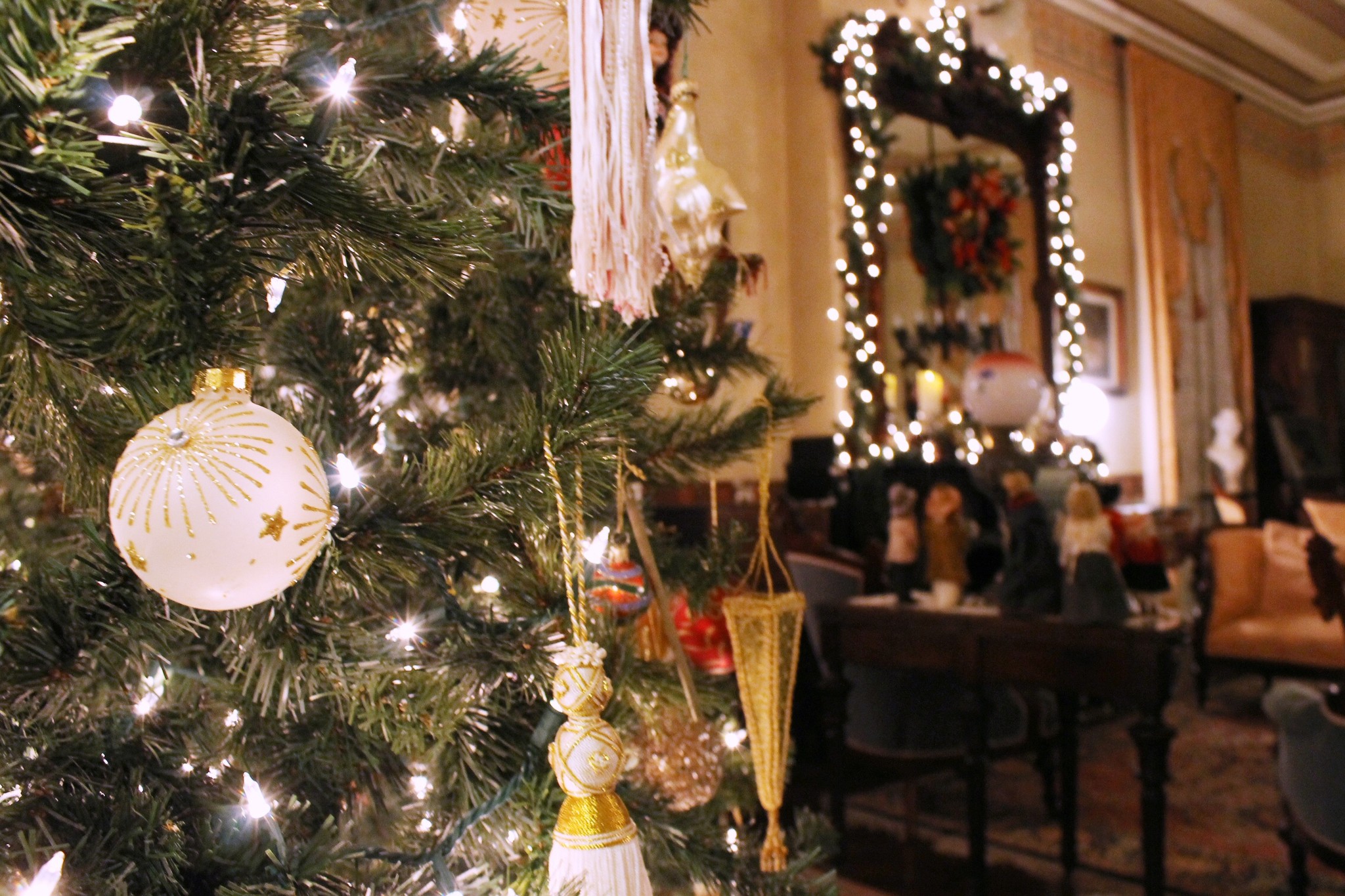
[819,1,1089,473]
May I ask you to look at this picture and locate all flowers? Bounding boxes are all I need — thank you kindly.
[897,144,1030,313]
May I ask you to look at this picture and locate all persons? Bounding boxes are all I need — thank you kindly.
[1203,409,1249,493]
[1056,483,1125,625]
[999,470,1055,610]
[885,484,924,609]
[918,482,980,609]
[827,417,1168,590]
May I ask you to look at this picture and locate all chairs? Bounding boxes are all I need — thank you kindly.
[1258,676,1345,896]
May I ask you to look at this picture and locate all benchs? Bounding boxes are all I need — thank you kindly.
[1184,505,1345,716]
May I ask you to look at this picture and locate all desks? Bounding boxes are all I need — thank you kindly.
[823,584,1187,896]
